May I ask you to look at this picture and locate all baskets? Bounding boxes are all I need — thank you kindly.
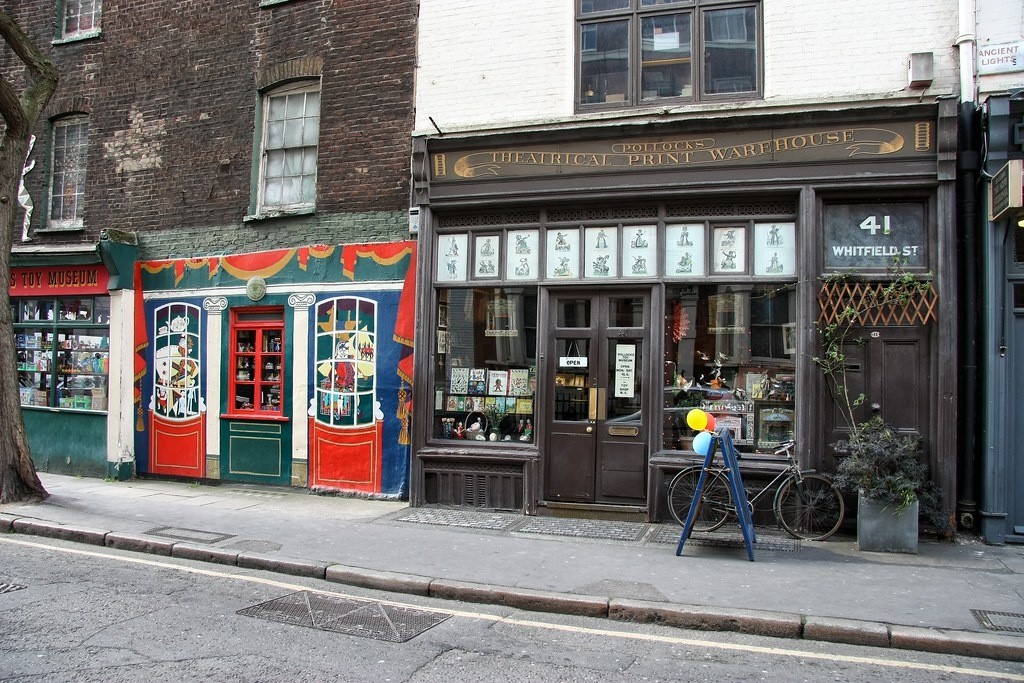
[463,410,488,440]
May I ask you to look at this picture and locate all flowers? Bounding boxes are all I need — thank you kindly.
[479,403,511,427]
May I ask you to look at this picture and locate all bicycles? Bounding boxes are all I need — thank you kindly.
[667,439,845,540]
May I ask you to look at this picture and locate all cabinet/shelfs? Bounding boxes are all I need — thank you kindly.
[15,346,52,374]
[57,347,108,376]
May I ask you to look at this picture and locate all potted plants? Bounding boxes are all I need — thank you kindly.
[747,252,960,556]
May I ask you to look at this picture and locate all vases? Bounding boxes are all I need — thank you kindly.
[490,428,500,441]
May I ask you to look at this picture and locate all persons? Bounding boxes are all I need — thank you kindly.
[443,418,465,439]
[518,419,532,440]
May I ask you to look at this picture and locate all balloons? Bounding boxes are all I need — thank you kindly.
[686,408,716,456]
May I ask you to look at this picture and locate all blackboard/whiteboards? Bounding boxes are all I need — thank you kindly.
[684,427,754,546]
